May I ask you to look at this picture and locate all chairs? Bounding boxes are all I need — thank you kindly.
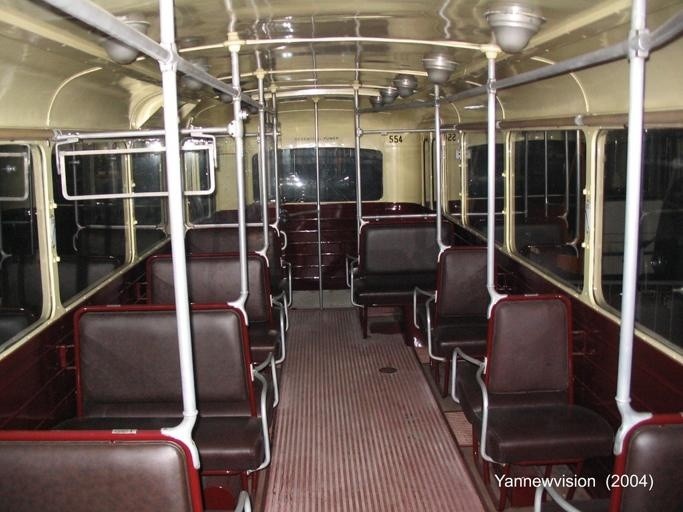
[428,246,680,510]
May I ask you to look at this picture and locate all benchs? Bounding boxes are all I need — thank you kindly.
[1,203,289,510]
[202,203,455,339]
